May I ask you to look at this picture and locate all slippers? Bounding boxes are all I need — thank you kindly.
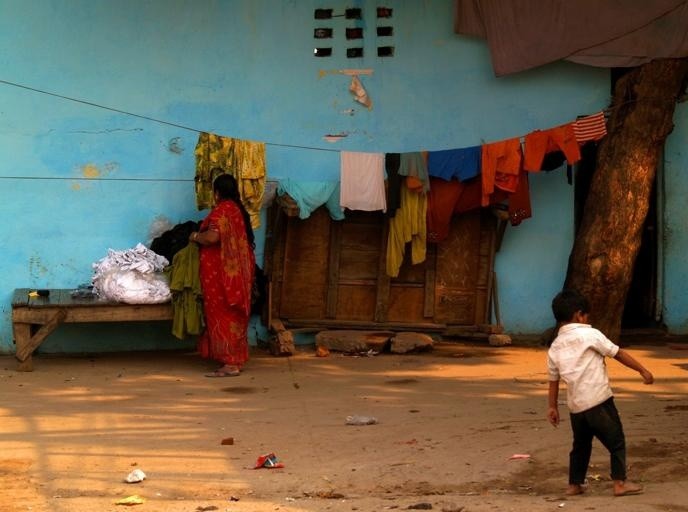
[204,369,241,377]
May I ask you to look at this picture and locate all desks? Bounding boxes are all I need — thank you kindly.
[12,287,178,373]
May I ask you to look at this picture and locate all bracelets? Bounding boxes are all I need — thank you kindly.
[193,231,200,242]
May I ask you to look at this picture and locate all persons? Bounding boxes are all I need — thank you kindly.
[547,290,654,497]
[188,173,256,378]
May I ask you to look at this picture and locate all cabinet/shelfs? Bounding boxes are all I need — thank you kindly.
[266,191,503,336]
